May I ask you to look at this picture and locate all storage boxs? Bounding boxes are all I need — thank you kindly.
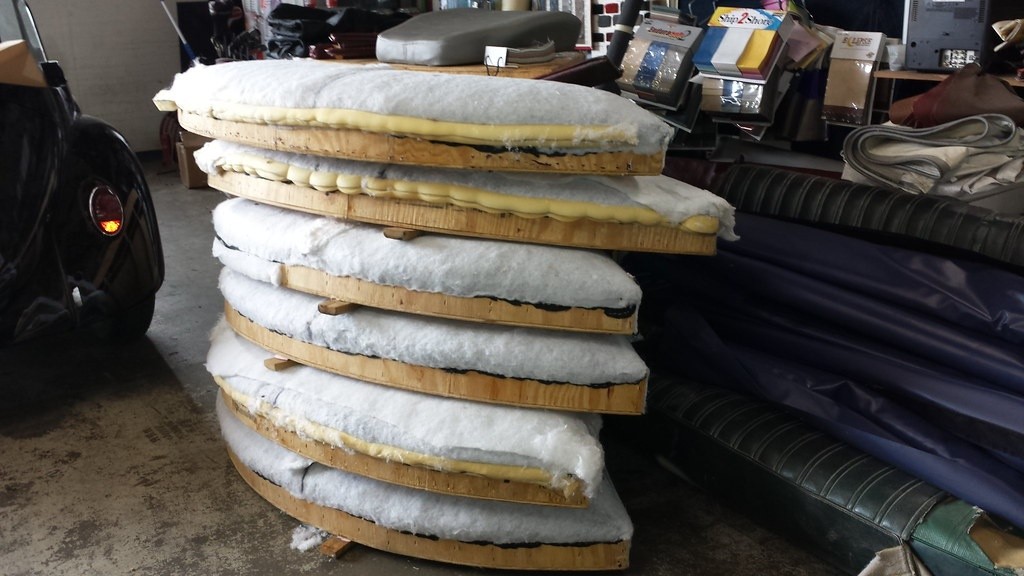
[175,131,209,188]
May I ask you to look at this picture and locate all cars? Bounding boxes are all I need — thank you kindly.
[0,1,165,370]
